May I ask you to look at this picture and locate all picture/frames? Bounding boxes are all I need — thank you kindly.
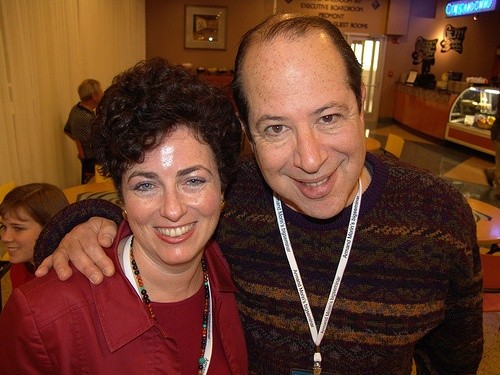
[183,3,228,52]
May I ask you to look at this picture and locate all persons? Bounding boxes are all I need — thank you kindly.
[0,58,249,375]
[1,183,69,292]
[37,14,484,375]
[484,92,500,199]
[64,79,103,185]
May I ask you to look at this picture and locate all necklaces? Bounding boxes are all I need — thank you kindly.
[130,235,210,375]
